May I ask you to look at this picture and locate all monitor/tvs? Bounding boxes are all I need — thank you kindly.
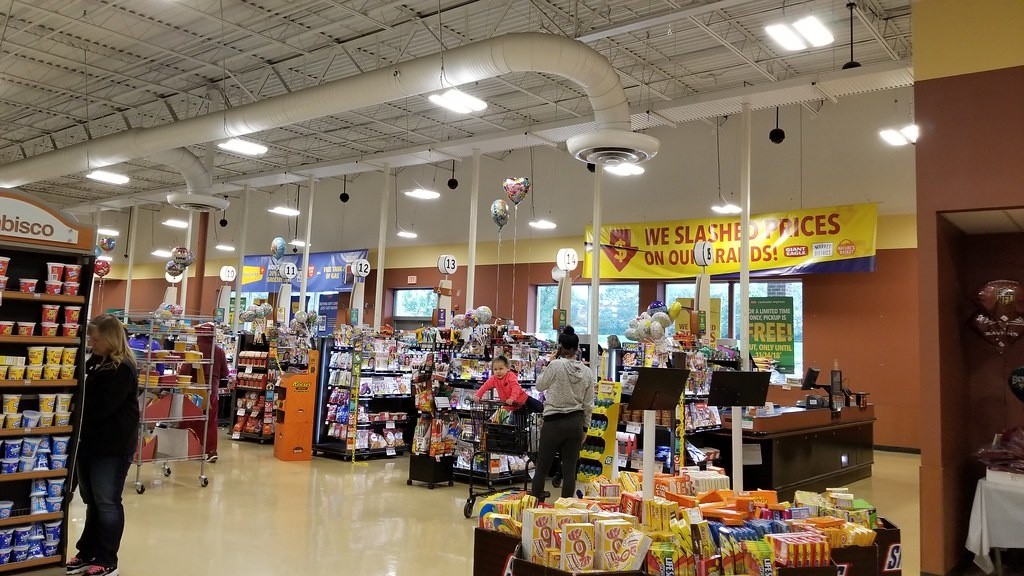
[801,367,820,390]
[831,369,842,395]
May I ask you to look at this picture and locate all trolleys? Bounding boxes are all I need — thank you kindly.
[464,396,551,518]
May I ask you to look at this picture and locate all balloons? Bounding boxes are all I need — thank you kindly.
[971,278,1024,353]
[490,200,510,229]
[166,260,185,276]
[289,310,322,335]
[503,178,530,204]
[172,245,196,267]
[154,302,184,326]
[240,303,274,321]
[624,301,682,342]
[270,237,285,260]
[94,244,101,258]
[451,305,492,328]
[99,235,116,250]
[94,259,110,277]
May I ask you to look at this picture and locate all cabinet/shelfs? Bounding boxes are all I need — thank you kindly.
[227,332,275,444]
[0,187,98,576]
[674,393,723,437]
[113,310,217,495]
[273,349,319,461]
[311,336,413,462]
[577,379,622,483]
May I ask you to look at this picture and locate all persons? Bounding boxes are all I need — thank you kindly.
[284,351,290,360]
[473,355,544,418]
[66,311,141,576]
[607,335,622,349]
[529,324,595,501]
[178,321,229,464]
[404,356,409,366]
[128,321,166,396]
[362,383,370,394]
[400,384,409,394]
[368,357,374,368]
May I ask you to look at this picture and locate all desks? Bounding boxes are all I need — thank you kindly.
[964,475,1024,576]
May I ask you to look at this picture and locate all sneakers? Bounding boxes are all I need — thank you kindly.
[65,555,96,574]
[82,560,119,576]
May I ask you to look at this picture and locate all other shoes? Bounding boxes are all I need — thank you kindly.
[206,452,219,463]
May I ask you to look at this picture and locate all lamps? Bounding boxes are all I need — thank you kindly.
[82,0,918,257]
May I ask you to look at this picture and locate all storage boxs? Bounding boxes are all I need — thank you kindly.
[152,427,203,460]
[986,466,1024,488]
[356,376,411,450]
[139,391,172,418]
[236,351,269,390]
[133,435,156,463]
[169,393,203,419]
[478,342,879,576]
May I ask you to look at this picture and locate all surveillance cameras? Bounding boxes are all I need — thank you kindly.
[340,193,349,202]
[448,179,458,189]
[587,163,596,173]
[124,255,128,258]
[220,219,227,227]
[769,128,785,144]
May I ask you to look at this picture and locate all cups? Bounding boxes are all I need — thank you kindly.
[0,256,81,565]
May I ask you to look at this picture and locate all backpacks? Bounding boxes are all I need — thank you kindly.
[136,337,157,371]
